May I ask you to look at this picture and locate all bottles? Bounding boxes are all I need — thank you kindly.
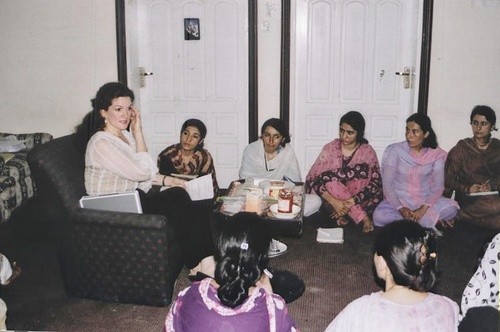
[277,190,295,213]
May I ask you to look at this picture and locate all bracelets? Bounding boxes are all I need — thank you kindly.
[163,175,167,186]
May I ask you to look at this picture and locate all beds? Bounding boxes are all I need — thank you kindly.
[0,132,54,225]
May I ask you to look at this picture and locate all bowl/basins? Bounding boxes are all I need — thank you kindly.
[270,203,301,220]
[259,178,294,198]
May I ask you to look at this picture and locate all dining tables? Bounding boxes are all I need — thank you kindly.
[215,178,307,304]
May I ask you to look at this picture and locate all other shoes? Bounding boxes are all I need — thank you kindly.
[188,270,213,282]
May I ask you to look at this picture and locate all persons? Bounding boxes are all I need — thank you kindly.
[238,118,304,236]
[71,82,289,282]
[303,111,384,235]
[157,118,250,235]
[458,231,500,332]
[373,112,461,239]
[186,212,275,295]
[0,251,27,332]
[164,212,297,332]
[322,219,460,332]
[444,106,500,233]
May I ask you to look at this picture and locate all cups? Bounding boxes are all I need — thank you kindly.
[246,191,261,213]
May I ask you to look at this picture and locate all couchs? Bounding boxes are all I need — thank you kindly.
[27,133,184,306]
